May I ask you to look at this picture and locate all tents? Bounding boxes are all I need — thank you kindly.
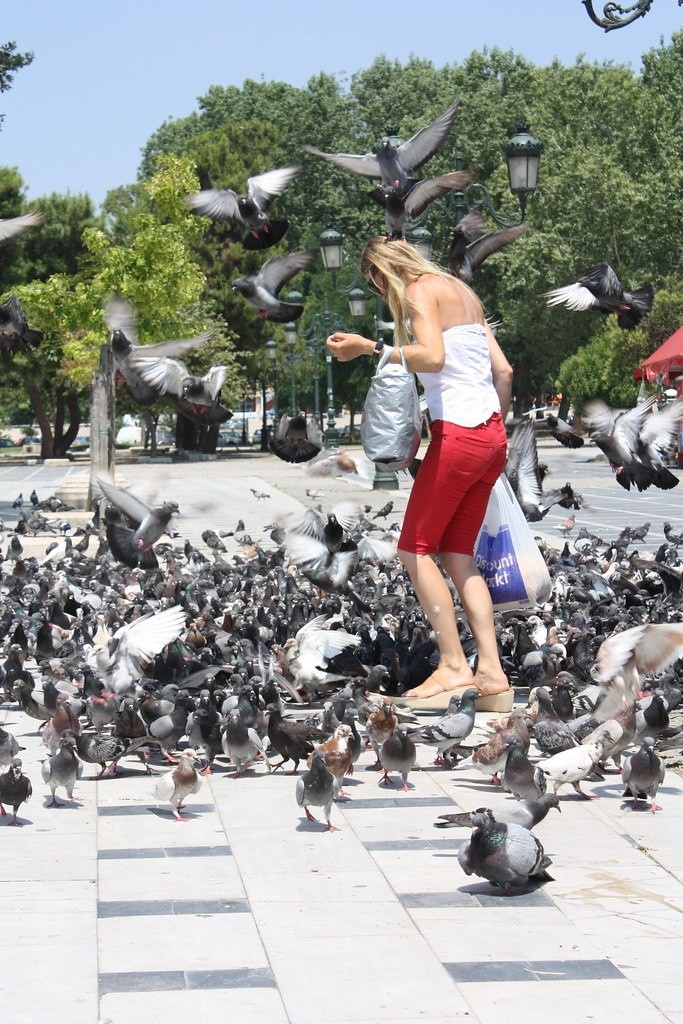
[634,323,682,469]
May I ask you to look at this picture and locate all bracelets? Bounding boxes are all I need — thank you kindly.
[371,337,385,362]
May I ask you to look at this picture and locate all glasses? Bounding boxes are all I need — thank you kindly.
[367,266,380,293]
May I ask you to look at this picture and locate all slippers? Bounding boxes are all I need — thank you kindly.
[389,671,476,710]
[475,675,515,712]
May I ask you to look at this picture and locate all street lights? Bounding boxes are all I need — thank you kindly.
[381,124,545,246]
[247,335,316,451]
[312,226,437,345]
[287,282,368,449]
[284,319,330,429]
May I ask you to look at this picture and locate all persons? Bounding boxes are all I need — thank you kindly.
[325,235,516,712]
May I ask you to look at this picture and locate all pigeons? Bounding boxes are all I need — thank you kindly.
[231,250,315,324]
[539,262,655,331]
[456,813,556,895]
[0,397,682,829]
[364,170,470,243]
[448,209,531,285]
[1,294,45,357]
[103,297,234,428]
[302,94,463,193]
[180,166,302,250]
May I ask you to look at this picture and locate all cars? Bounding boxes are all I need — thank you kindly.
[0,406,288,447]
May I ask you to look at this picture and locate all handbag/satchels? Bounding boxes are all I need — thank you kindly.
[359,343,422,472]
[473,470,552,611]
